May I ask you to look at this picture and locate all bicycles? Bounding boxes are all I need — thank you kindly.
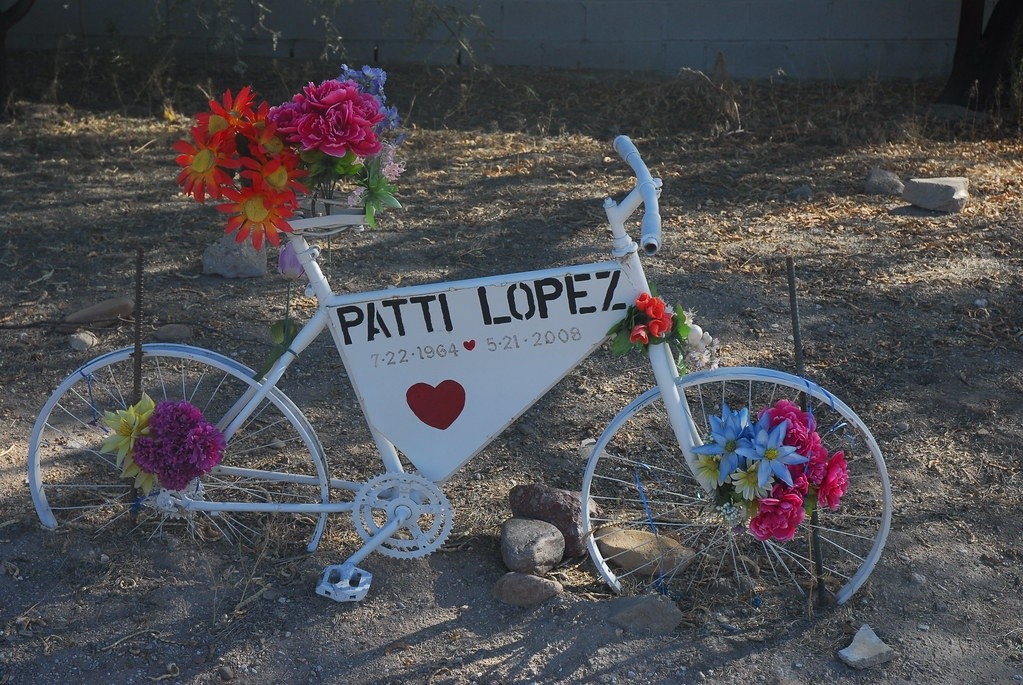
[27,134,892,618]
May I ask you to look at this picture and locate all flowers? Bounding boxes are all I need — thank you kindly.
[97,391,227,498]
[171,60,407,252]
[269,243,308,351]
[690,400,850,541]
[612,291,715,380]
